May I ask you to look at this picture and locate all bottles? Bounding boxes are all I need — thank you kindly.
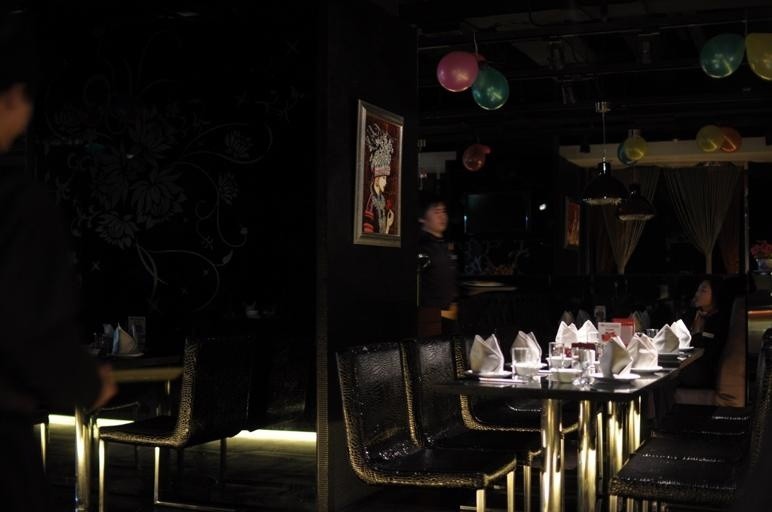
[594,305,607,328]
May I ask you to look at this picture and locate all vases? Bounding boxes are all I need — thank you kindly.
[756,259,772,275]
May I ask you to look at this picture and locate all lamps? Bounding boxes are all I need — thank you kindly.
[581,96,655,222]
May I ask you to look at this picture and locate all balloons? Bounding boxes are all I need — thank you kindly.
[473,69,510,111]
[698,125,723,151]
[437,50,478,92]
[461,143,485,170]
[699,32,745,78]
[617,142,636,167]
[721,128,743,153]
[746,34,772,79]
[624,134,649,161]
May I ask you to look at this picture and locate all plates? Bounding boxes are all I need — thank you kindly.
[466,345,695,382]
[110,351,145,358]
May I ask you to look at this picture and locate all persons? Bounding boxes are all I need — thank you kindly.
[361,119,398,235]
[0,49,120,511]
[653,274,726,422]
[415,194,458,333]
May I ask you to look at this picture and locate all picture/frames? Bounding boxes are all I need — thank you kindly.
[349,94,408,253]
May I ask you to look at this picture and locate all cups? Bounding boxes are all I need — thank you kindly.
[586,331,599,343]
[646,329,658,337]
[548,341,565,369]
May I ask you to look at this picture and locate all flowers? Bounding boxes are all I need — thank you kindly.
[750,239,772,260]
